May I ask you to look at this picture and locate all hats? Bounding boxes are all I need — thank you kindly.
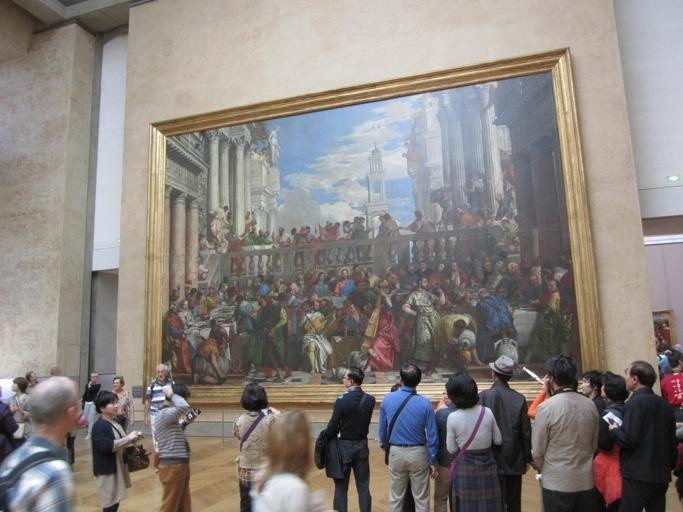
[488,355,515,377]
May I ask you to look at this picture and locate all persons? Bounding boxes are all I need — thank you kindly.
[528,344,683,512]
[379,355,533,512]
[233,382,326,511]
[322,366,375,511]
[1,366,198,512]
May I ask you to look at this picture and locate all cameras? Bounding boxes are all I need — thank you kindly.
[261,409,270,416]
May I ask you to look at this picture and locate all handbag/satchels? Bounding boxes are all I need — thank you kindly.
[122,444,152,474]
[445,460,458,480]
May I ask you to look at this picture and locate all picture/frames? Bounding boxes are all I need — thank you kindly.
[142,47,607,406]
[653,309,676,354]
